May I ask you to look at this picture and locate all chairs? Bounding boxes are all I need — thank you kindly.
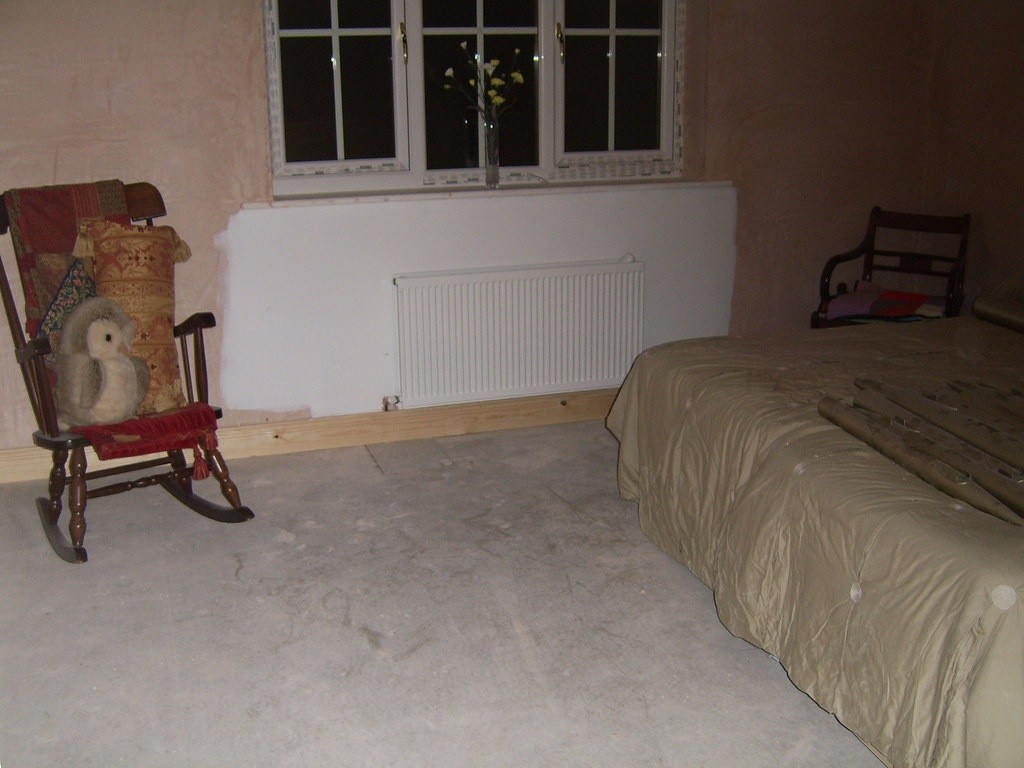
[809,205,970,327]
[0,176,254,561]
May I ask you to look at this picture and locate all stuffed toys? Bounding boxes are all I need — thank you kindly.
[55,295,147,431]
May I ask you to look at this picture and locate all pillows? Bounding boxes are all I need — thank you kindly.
[74,212,192,416]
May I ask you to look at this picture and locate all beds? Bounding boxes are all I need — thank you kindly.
[603,317,1024,768]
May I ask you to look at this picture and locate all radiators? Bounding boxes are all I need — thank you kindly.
[385,257,645,412]
